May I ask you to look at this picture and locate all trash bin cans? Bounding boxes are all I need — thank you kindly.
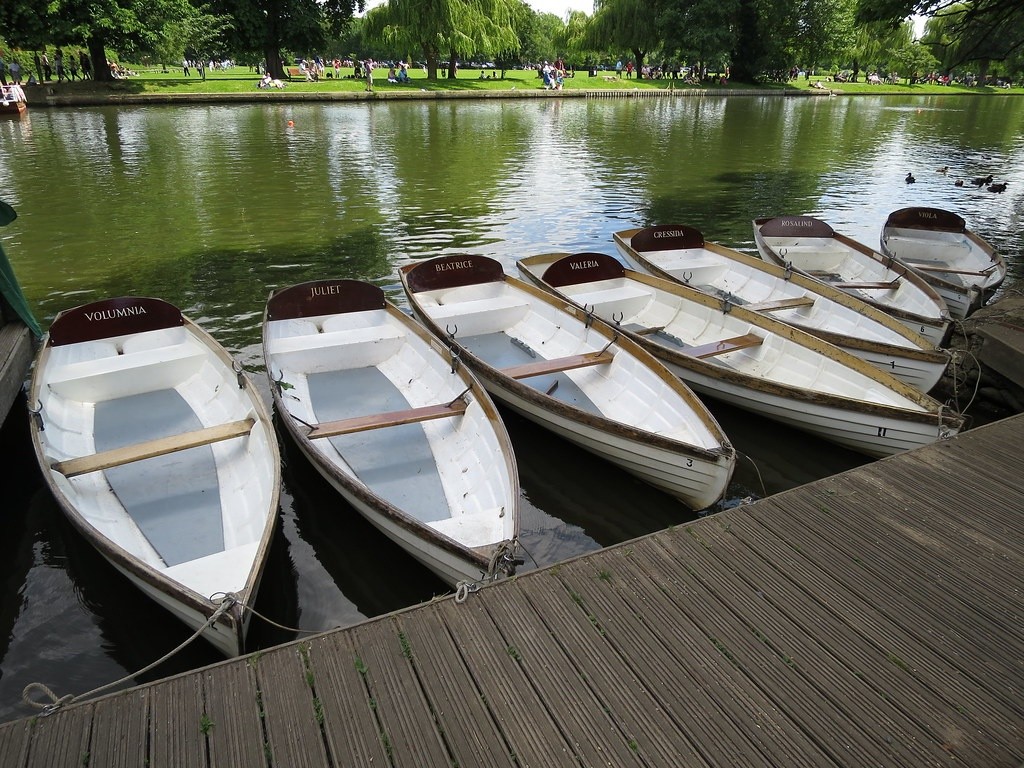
[588,66,597,77]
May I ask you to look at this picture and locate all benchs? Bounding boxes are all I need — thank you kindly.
[49,342,204,403]
[741,295,814,315]
[268,324,407,375]
[826,278,902,288]
[423,295,529,338]
[680,334,763,361]
[887,234,968,262]
[287,68,318,82]
[656,256,727,286]
[50,417,255,478]
[566,285,652,322]
[499,351,613,379]
[299,399,467,439]
[908,261,992,278]
[769,245,847,271]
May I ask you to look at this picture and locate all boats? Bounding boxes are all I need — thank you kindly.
[27,296,278,659]
[0,84,27,114]
[876,206,1008,320]
[514,248,966,458]
[399,253,739,515]
[261,277,522,596]
[749,215,959,350]
[611,223,962,399]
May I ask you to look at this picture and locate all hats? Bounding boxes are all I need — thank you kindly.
[311,60,315,63]
[302,60,306,63]
[111,66,115,68]
[874,73,877,75]
[550,67,554,71]
[870,74,872,76]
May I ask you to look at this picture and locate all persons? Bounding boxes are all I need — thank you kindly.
[250,57,575,92]
[641,59,729,88]
[183,58,235,77]
[616,60,633,80]
[0,50,140,86]
[790,65,1011,90]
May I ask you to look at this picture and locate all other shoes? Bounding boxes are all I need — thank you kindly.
[90,77,92,79]
[369,89,372,91]
[189,74,190,76]
[185,76,186,78]
[690,84,691,85]
[67,80,71,83]
[58,80,61,83]
[87,78,90,80]
[83,78,84,80]
[365,89,369,91]
[46,79,51,81]
[310,79,314,81]
[80,80,83,82]
